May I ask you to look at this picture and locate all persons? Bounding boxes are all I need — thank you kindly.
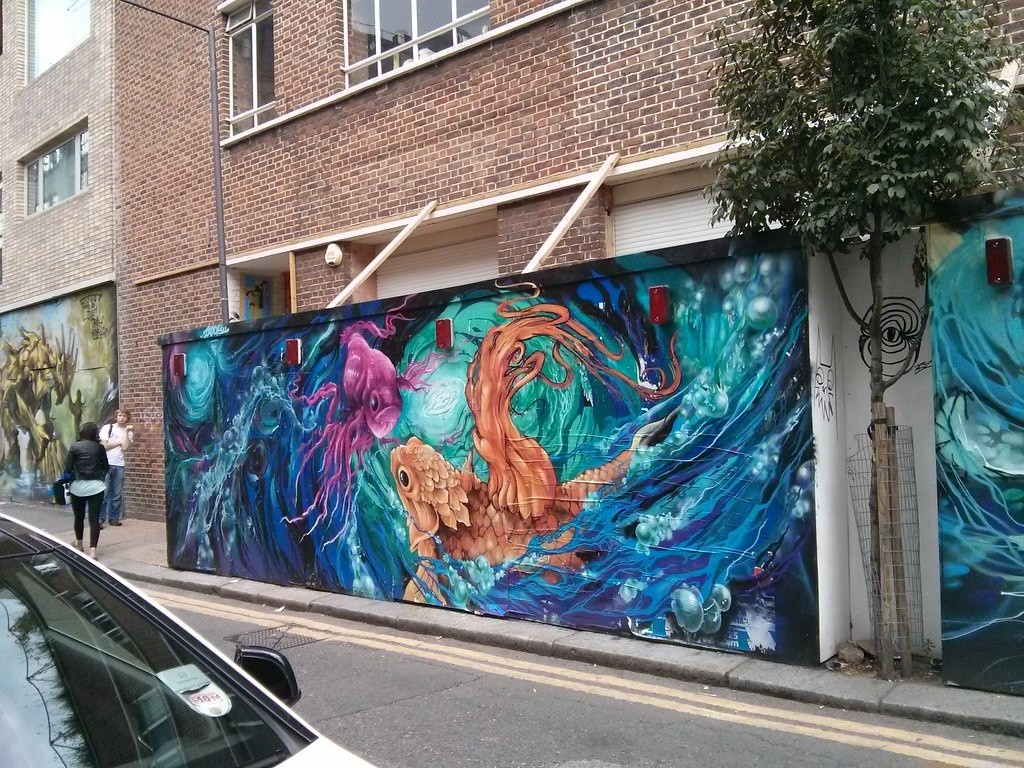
[99,408,134,528]
[61,420,110,559]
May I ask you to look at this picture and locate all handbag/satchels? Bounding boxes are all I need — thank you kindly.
[53,478,72,505]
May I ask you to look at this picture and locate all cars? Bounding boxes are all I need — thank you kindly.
[0,513,380,768]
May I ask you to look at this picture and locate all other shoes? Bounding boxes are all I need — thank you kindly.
[71,540,84,552]
[87,547,99,561]
[108,519,122,526]
[98,520,104,529]
